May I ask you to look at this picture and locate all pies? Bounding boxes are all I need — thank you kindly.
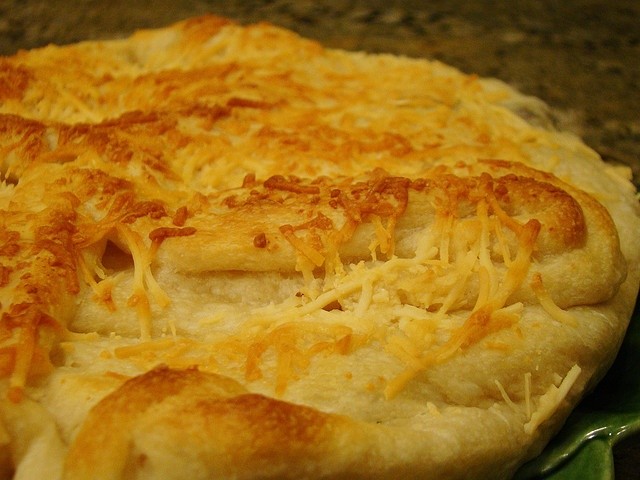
[0,13,640,480]
